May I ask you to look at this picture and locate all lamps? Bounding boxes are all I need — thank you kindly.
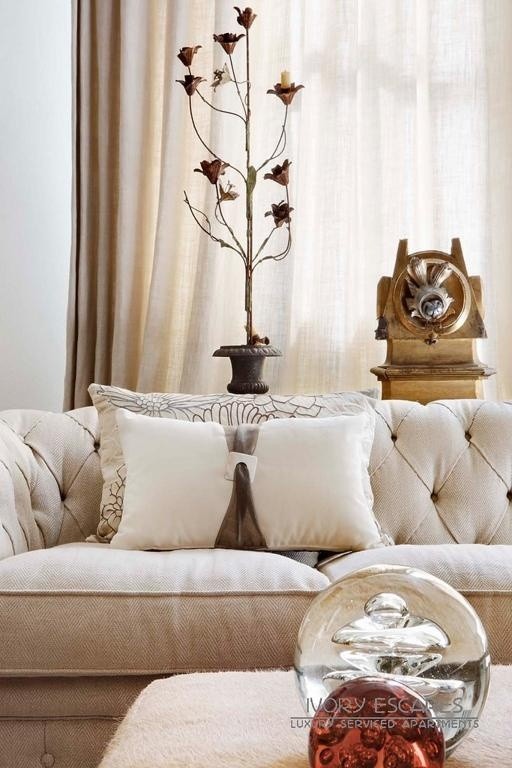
[174,6,306,394]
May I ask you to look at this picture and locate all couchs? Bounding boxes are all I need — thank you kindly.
[99,665,512,768]
[1,399,512,768]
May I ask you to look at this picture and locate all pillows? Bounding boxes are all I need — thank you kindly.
[85,381,398,570]
